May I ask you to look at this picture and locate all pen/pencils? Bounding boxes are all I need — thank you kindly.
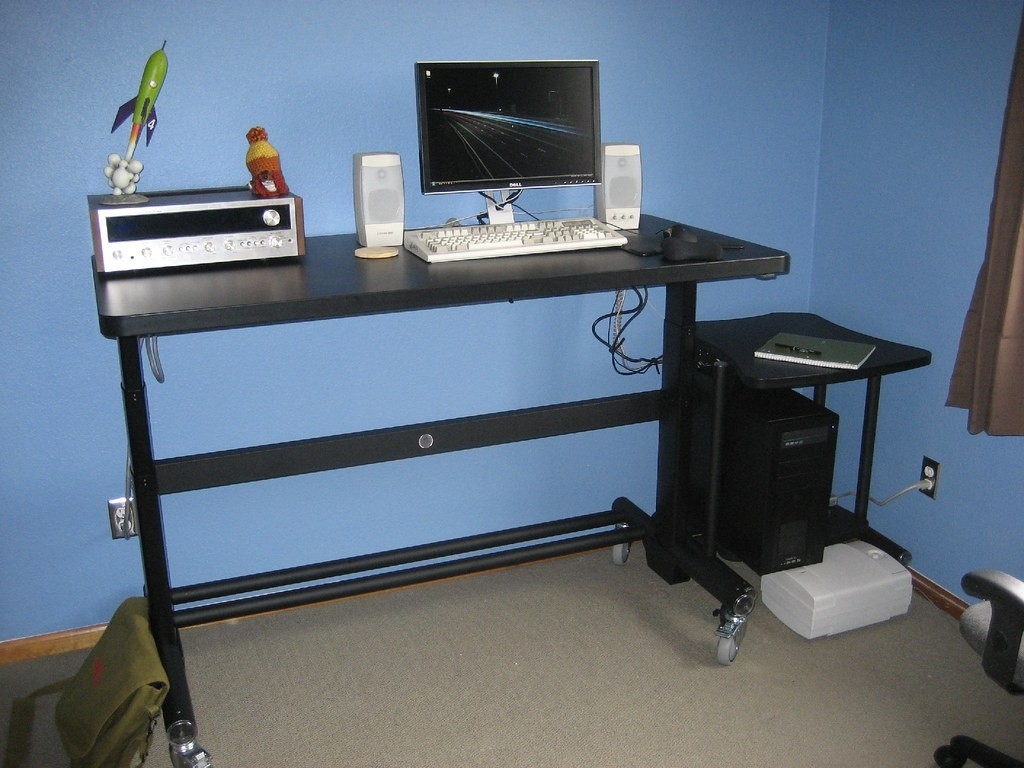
[722,244,744,250]
[775,342,822,354]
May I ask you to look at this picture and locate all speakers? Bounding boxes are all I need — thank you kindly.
[353,152,404,248]
[594,143,641,230]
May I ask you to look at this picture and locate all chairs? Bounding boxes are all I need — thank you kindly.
[934,567,1024,768]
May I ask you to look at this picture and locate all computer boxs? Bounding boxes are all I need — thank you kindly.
[685,368,840,578]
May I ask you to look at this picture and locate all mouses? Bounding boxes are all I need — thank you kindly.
[664,225,698,243]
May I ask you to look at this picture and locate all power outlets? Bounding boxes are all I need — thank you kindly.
[916,455,941,498]
[108,498,138,541]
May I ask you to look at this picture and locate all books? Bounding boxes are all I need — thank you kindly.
[754,333,876,369]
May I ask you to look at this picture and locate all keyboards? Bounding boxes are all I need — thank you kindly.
[402,217,628,263]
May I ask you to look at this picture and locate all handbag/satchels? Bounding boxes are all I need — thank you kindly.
[5,597,170,768]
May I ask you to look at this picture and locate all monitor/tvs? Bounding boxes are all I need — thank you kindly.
[415,59,603,226]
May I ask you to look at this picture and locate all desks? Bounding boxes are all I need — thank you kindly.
[89,213,933,768]
